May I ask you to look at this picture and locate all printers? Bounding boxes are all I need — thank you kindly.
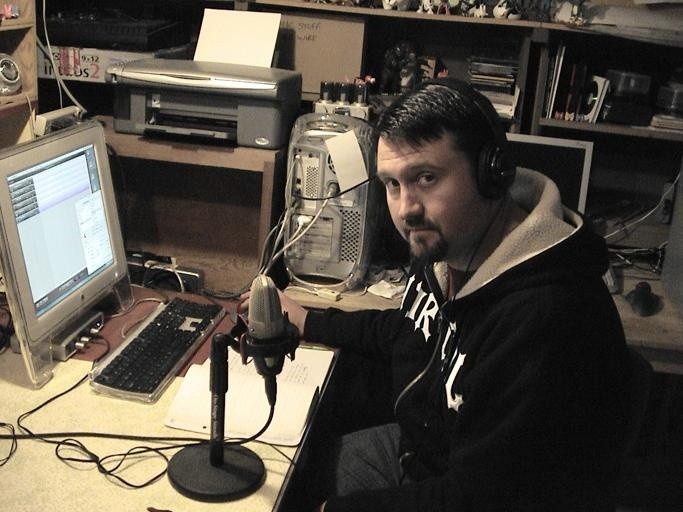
[104,49,303,150]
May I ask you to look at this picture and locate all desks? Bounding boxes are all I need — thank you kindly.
[286,267,683,377]
[1,265,338,509]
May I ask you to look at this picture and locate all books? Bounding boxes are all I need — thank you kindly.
[468,55,516,119]
[546,39,610,123]
[164,335,335,446]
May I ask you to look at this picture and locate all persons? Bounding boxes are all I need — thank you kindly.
[240,76,632,512]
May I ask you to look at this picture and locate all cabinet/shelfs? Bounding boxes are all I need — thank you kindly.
[0,0,37,115]
[231,1,681,248]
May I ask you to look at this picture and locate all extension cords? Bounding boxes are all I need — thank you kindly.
[33,105,84,136]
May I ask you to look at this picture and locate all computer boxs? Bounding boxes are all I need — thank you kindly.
[283,112,380,291]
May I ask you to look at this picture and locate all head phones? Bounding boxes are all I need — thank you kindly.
[417,75,519,201]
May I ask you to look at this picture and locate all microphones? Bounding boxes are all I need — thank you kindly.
[246,274,286,407]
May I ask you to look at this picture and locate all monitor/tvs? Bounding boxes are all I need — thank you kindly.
[506,131,595,215]
[0,118,129,390]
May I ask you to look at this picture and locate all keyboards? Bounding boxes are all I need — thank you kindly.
[602,265,620,293]
[87,296,226,403]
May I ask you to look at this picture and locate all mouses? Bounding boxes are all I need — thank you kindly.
[624,289,660,318]
